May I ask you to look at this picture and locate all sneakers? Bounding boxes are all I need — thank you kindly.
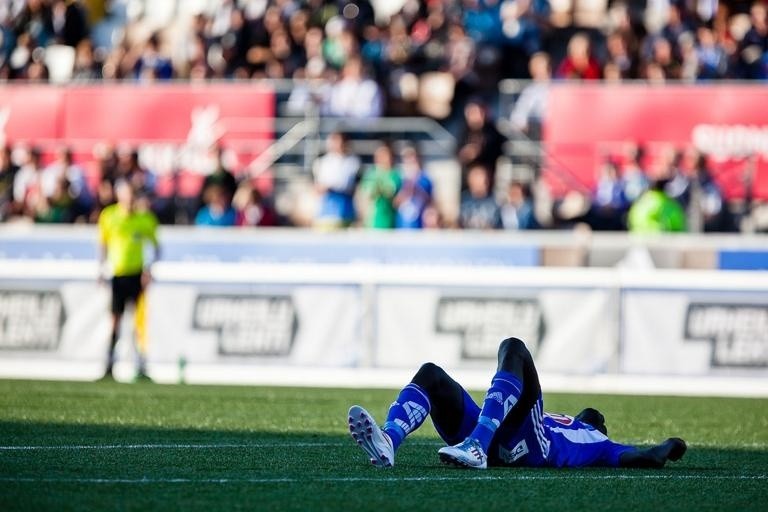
[438,436,489,470]
[347,404,395,468]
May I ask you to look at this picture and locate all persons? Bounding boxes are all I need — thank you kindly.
[347,335,688,470]
[0,1,767,237]
[96,177,161,382]
